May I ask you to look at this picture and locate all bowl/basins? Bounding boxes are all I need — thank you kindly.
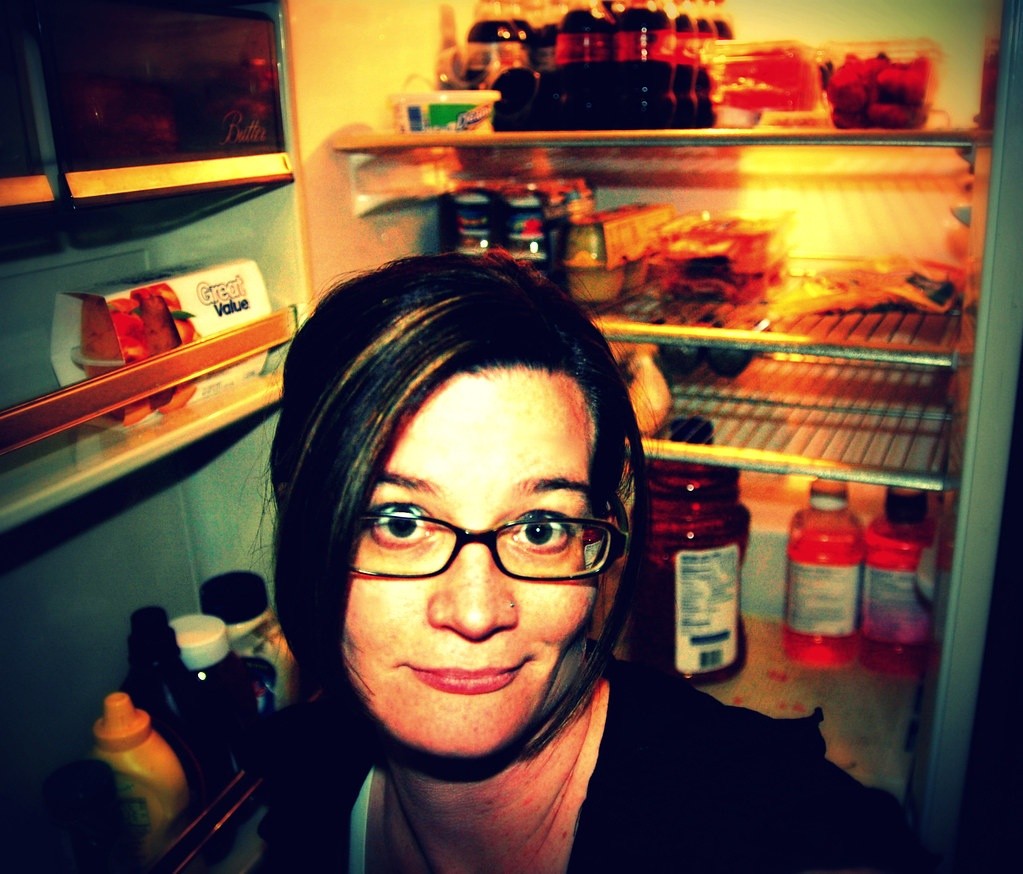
[700,35,941,131]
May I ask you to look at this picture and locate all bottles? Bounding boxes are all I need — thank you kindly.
[779,474,941,680]
[442,0,731,133]
[94,569,297,837]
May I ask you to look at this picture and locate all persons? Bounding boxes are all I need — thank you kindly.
[261,257,944,874]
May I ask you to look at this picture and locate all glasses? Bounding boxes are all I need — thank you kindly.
[346,512,630,581]
